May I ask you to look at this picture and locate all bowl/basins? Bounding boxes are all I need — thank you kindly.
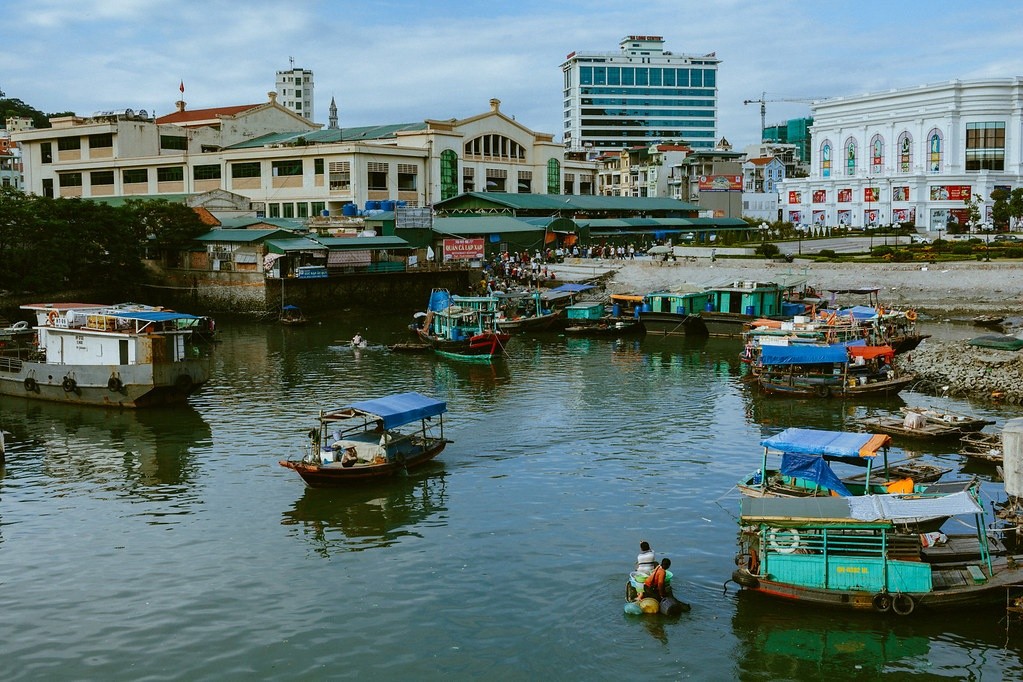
[638,598,659,613]
[624,603,644,615]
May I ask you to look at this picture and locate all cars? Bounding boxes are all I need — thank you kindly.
[909,233,933,246]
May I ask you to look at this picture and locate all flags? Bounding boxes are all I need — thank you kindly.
[179,79,185,93]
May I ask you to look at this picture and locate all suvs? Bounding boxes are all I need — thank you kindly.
[994,234,1023,243]
[951,234,986,243]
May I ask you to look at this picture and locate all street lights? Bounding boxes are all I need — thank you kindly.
[795,224,805,255]
[934,223,945,256]
[886,177,895,225]
[892,223,902,249]
[865,176,875,253]
[758,222,770,246]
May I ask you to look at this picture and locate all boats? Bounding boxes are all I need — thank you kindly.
[0,301,216,409]
[714,425,1023,616]
[484,277,1005,463]
[623,569,690,621]
[406,287,515,359]
[277,390,455,488]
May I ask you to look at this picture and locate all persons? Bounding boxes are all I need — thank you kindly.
[426,243,435,272]
[653,561,659,569]
[638,557,671,601]
[636,540,655,573]
[711,248,717,262]
[281,309,301,321]
[352,332,364,345]
[485,237,680,263]
[476,259,555,319]
[341,442,358,468]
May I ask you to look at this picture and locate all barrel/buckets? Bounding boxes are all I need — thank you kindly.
[343,199,406,217]
[452,328,461,340]
[677,306,685,315]
[331,430,342,442]
[659,597,682,617]
[705,302,713,312]
[642,304,650,312]
[613,304,622,317]
[634,305,641,318]
[746,306,754,315]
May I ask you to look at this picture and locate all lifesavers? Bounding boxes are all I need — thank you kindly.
[904,310,918,320]
[23,378,36,391]
[769,527,800,553]
[62,378,77,392]
[177,373,193,393]
[48,310,60,325]
[816,384,829,398]
[872,593,915,616]
[107,377,122,392]
[393,452,408,467]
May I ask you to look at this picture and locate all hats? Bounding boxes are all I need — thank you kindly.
[344,442,356,449]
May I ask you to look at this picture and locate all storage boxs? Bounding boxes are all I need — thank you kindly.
[630,569,673,593]
[794,316,810,324]
[759,336,789,347]
[469,258,483,268]
[780,322,794,331]
[855,356,866,366]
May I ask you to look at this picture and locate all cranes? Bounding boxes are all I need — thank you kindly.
[743,89,832,130]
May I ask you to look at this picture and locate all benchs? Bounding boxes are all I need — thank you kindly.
[967,565,987,585]
